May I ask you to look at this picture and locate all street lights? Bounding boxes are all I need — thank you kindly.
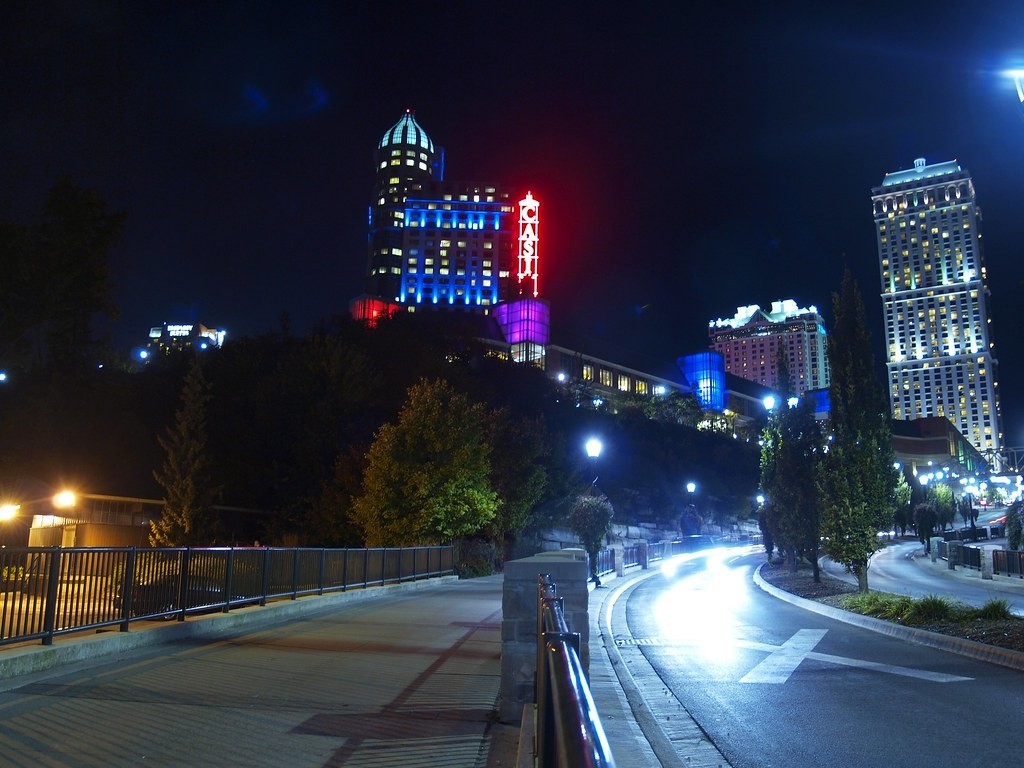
[686,483,696,510]
[585,438,602,581]
